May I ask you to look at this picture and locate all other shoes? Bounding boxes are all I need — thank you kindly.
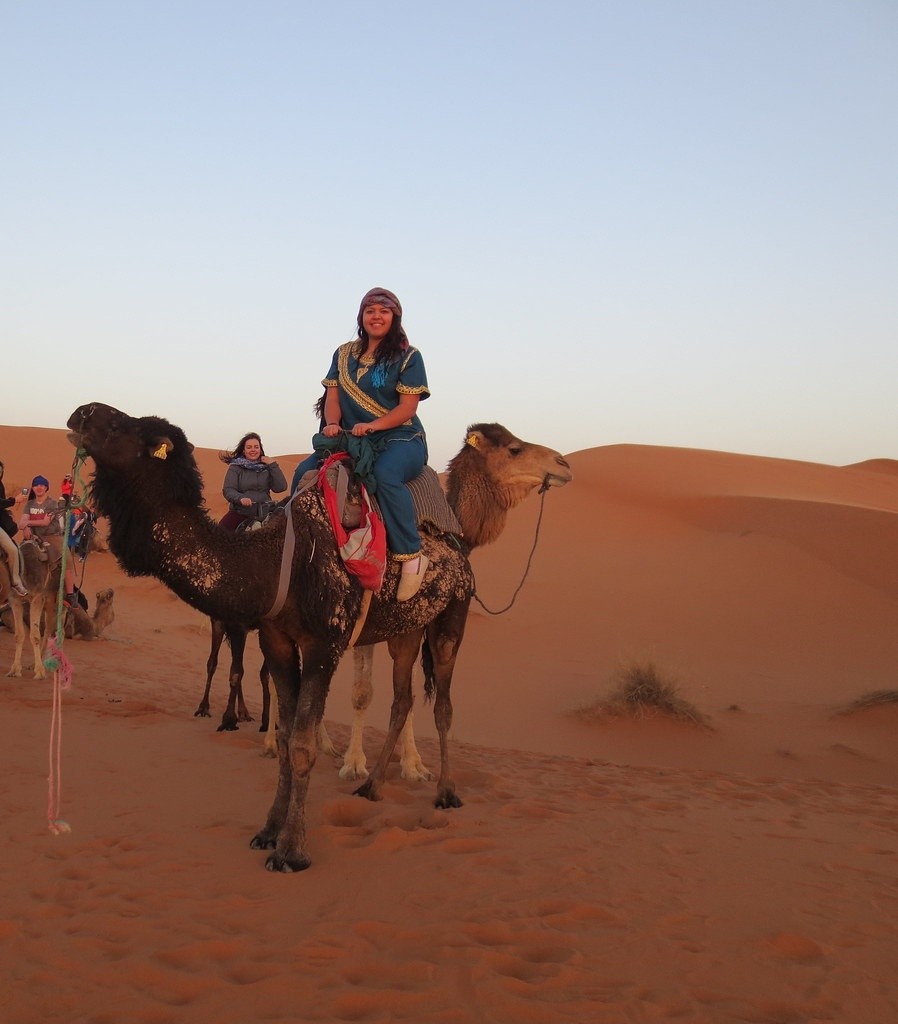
[63,593,79,610]
[397,553,429,601]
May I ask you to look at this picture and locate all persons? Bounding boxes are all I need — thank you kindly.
[291,288,432,602]
[218,431,288,533]
[0,462,29,597]
[61,474,75,506]
[18,475,80,610]
[84,504,97,527]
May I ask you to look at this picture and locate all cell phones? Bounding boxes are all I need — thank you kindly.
[22,488,29,495]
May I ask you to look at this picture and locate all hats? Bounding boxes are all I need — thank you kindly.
[357,288,402,325]
[32,475,48,486]
[64,474,72,479]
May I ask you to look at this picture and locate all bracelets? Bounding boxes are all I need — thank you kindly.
[328,423,337,425]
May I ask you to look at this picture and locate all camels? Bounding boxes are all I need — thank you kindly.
[58,497,111,553]
[65,402,572,874]
[0,533,115,680]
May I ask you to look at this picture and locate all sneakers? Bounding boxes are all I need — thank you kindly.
[11,584,28,596]
